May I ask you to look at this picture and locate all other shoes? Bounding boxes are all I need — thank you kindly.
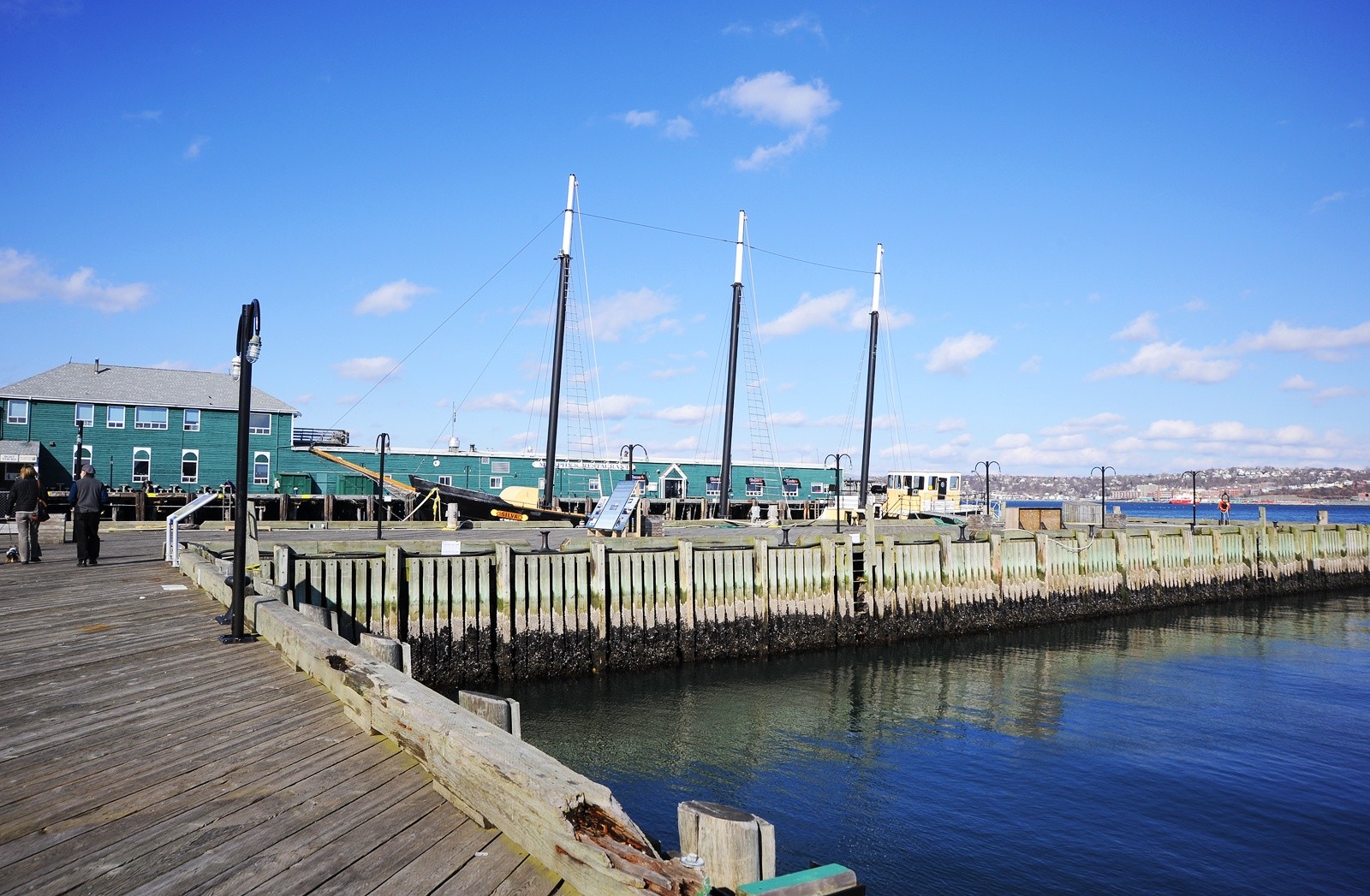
[89,560,97,564]
[77,559,87,566]
[30,556,41,561]
[21,561,28,565]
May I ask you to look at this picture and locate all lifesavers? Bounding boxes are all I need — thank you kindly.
[1219,501,1231,512]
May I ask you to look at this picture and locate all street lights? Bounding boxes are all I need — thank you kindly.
[823,453,854,533]
[463,465,471,488]
[110,455,114,489]
[74,420,85,481]
[975,461,1001,514]
[557,469,566,498]
[219,299,264,645]
[784,475,794,500]
[620,443,649,533]
[1181,471,1208,526]
[1090,466,1117,528]
[375,433,392,540]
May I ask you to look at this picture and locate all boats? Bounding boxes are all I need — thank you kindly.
[1170,492,1203,506]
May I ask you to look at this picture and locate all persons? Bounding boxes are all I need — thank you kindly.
[274,479,280,494]
[68,465,107,568]
[5,465,50,565]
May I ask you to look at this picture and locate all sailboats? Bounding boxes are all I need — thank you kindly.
[307,173,969,521]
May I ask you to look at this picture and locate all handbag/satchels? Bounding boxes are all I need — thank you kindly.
[32,498,50,522]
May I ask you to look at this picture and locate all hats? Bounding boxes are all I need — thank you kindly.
[82,464,95,474]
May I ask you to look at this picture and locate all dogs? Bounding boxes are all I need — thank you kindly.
[5,545,20,563]
